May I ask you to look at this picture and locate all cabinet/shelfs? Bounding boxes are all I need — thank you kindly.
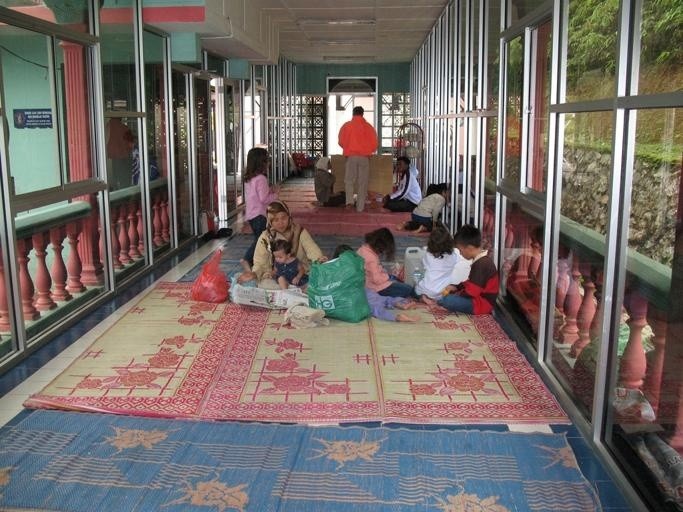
[396,124,423,193]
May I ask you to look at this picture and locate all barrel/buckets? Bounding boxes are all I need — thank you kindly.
[301,167,313,178]
[404,246,428,288]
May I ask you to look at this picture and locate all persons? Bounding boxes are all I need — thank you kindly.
[411,228,458,304]
[422,223,501,317]
[107,118,158,191]
[238,198,328,290]
[268,239,308,295]
[339,106,379,212]
[239,148,280,273]
[355,228,414,298]
[333,244,419,322]
[382,156,422,212]
[313,156,345,206]
[394,183,451,234]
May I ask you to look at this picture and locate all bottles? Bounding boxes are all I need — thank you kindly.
[411,267,422,288]
